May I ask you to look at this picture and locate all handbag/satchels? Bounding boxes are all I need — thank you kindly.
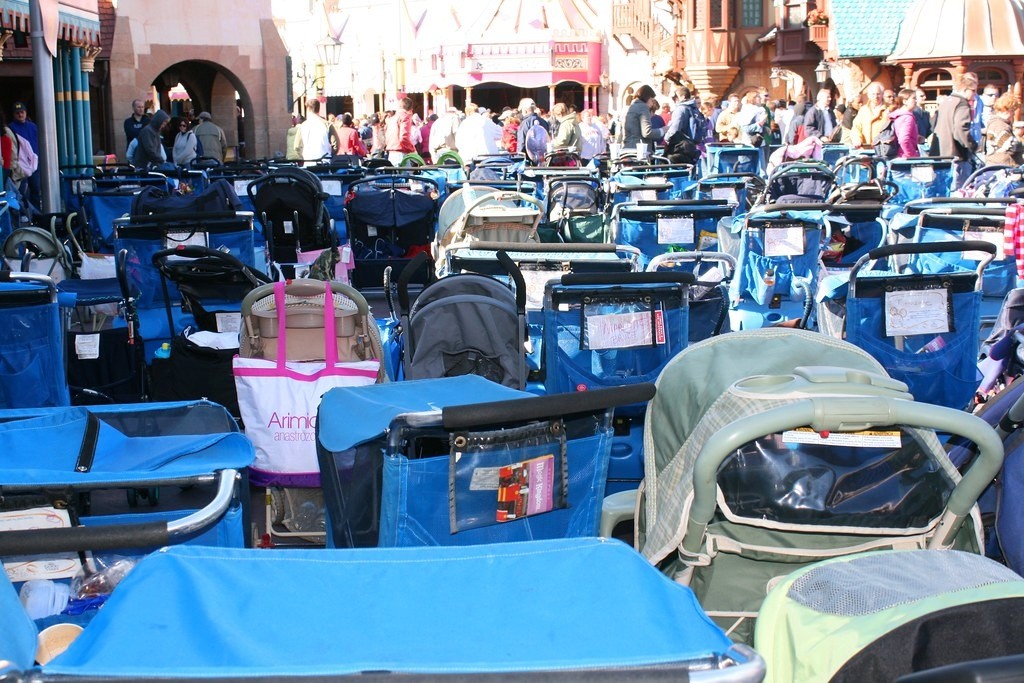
[232,280,380,487]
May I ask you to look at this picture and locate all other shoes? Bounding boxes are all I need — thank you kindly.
[20,216,27,223]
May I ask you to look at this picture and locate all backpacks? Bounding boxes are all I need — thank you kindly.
[16,134,37,177]
[870,114,911,159]
[187,133,203,156]
[681,105,709,141]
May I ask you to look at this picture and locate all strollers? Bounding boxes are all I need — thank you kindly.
[1,139,1024,682]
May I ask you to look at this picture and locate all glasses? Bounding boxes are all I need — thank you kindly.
[967,87,976,93]
[986,93,999,97]
[180,123,187,127]
[760,94,768,97]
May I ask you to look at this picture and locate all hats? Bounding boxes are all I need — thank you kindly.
[13,102,27,113]
[498,110,515,120]
[518,98,536,111]
[195,112,211,119]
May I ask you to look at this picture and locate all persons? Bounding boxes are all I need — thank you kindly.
[517,97,552,164]
[125,98,245,172]
[385,97,417,167]
[455,103,503,165]
[429,106,461,164]
[578,109,601,167]
[173,118,199,171]
[622,85,670,153]
[286,70,1024,169]
[0,100,41,223]
[293,99,338,166]
[551,103,583,159]
[192,113,227,166]
